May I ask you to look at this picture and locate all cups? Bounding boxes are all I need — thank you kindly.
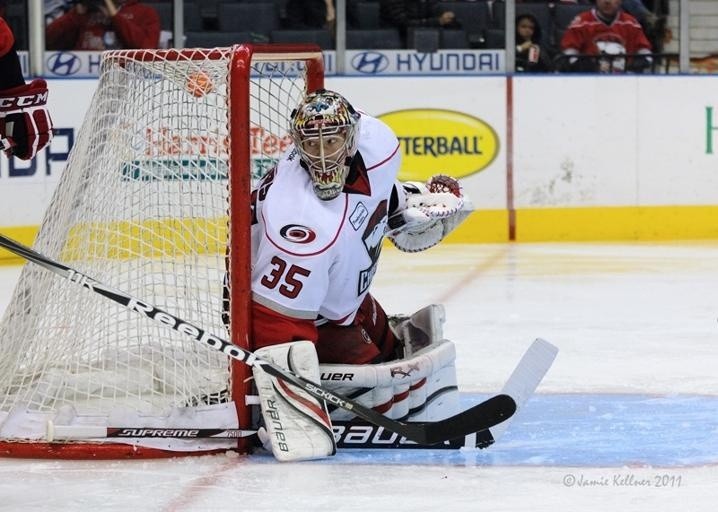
[528,46,541,64]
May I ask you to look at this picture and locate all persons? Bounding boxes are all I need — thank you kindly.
[221,87,475,458]
[0,14,54,162]
[42,1,668,73]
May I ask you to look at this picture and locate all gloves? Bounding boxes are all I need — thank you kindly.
[0,78,53,160]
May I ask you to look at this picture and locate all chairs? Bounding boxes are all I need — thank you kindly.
[0,0,670,73]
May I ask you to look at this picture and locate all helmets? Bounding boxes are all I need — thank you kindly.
[289,89,360,200]
